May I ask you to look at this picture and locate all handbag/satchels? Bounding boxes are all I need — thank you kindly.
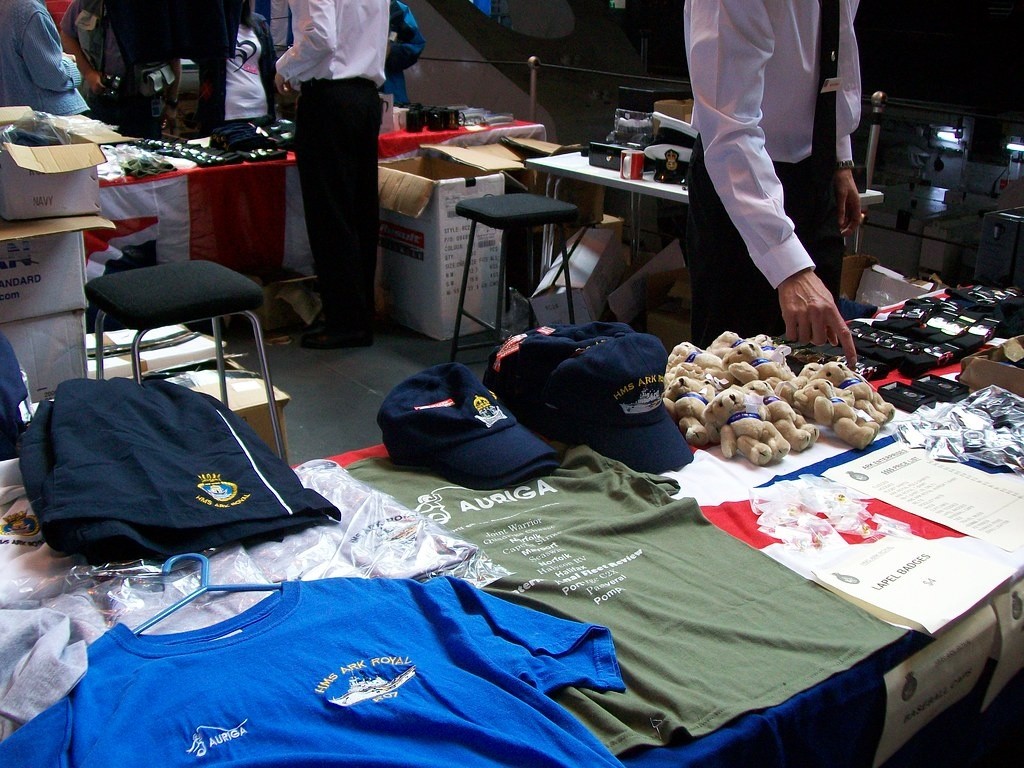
[139,61,176,96]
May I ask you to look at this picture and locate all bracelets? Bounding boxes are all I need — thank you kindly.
[836,160,854,170]
[165,99,178,108]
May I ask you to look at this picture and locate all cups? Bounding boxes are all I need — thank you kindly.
[391,101,466,133]
[619,150,645,181]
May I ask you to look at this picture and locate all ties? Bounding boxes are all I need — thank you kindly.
[810,0,840,194]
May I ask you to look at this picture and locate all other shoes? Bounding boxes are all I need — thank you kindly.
[300,326,374,350]
[229,315,293,346]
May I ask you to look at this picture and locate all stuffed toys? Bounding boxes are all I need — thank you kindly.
[663,331,895,467]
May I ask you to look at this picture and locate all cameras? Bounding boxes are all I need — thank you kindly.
[97,75,120,96]
[425,105,458,131]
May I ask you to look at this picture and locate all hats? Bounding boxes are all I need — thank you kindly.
[644,143,700,187]
[375,313,697,492]
[650,111,699,149]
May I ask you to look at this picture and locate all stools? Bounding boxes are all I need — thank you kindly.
[87,259,290,466]
[451,192,578,362]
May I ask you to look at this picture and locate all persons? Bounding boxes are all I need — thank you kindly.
[382,0,426,103]
[683,0,861,366]
[60,0,181,139]
[201,0,279,137]
[0,0,91,116]
[275,0,390,349]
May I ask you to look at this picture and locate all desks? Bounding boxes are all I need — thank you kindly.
[0,283,1024,768]
[82,137,319,287]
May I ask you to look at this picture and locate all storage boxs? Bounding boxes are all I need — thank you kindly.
[1,107,321,465]
[378,135,688,342]
[956,335,1024,398]
[835,255,940,318]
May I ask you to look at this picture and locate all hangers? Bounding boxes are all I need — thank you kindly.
[130,554,282,636]
